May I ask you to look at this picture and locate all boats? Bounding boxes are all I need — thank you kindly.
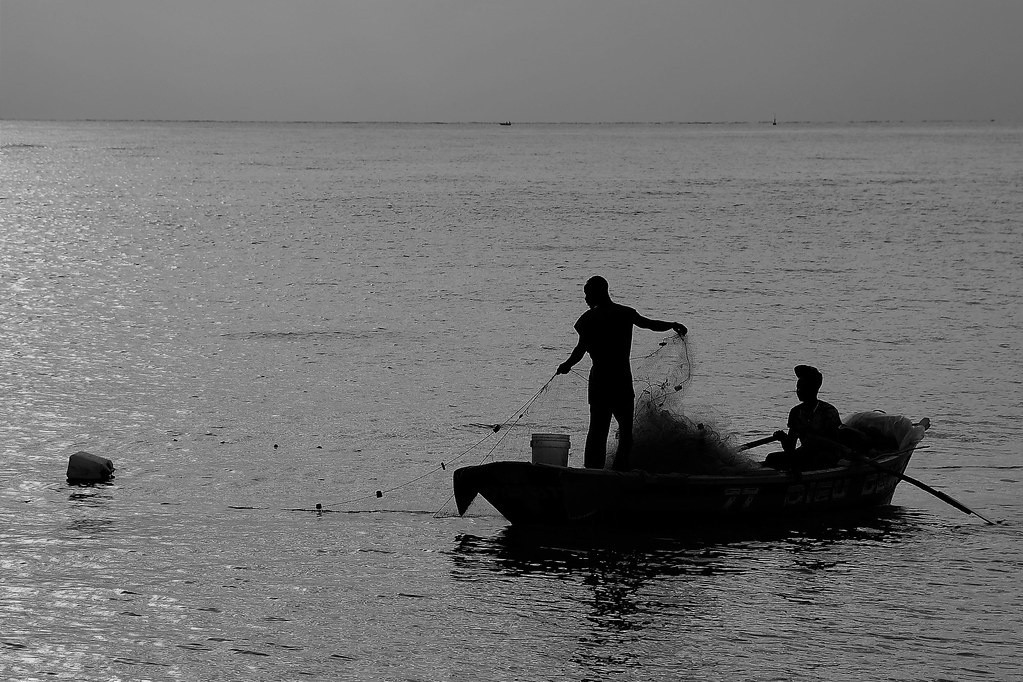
[452,417,929,543]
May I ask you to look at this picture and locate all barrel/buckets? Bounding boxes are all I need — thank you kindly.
[530,433,571,466]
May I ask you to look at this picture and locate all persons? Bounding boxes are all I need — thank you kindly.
[556,276,687,470]
[763,364,843,471]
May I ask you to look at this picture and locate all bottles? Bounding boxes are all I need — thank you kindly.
[67,451,115,477]
